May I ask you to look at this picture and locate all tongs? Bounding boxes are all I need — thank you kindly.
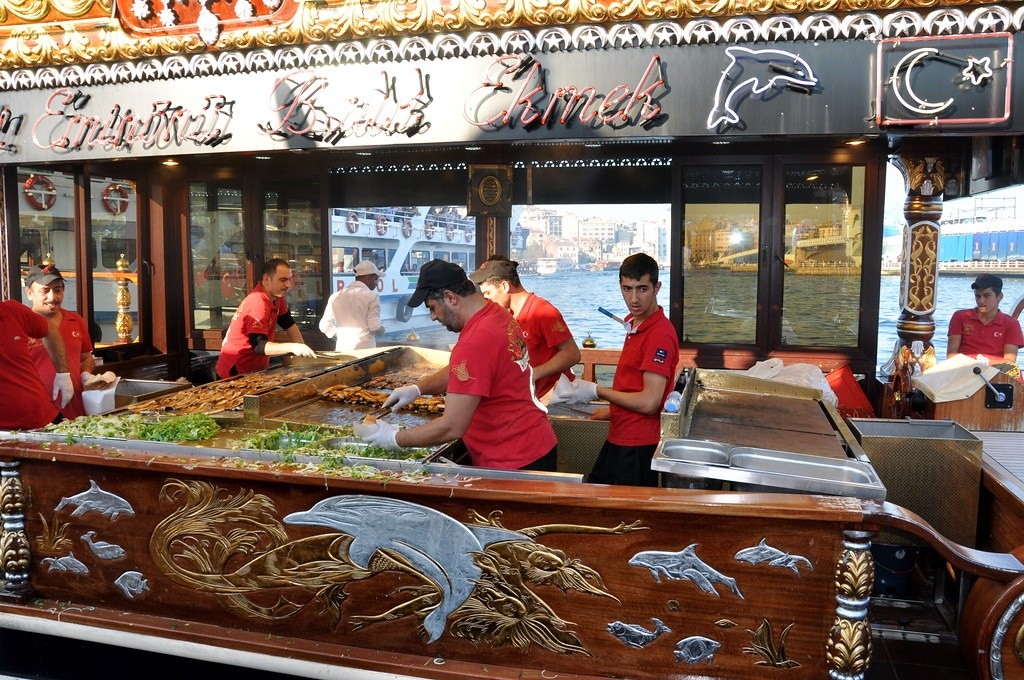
[357,406,392,421]
[315,351,342,360]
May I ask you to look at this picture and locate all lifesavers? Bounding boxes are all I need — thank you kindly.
[446,224,454,241]
[274,210,290,228]
[402,220,413,238]
[465,227,472,242]
[512,232,518,245]
[102,183,129,214]
[376,216,388,235]
[312,208,321,231]
[24,176,56,210]
[425,223,435,239]
[346,213,359,233]
[396,295,414,323]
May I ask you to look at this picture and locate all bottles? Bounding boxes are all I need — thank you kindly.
[664,391,682,412]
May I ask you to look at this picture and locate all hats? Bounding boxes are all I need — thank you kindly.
[973,274,1004,292]
[467,254,521,282]
[406,257,468,307]
[23,264,68,284]
[352,260,388,278]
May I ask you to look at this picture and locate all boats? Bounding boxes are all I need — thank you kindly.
[536,258,576,275]
[18,166,530,343]
[517,260,538,277]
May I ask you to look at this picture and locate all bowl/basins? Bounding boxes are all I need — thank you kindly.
[353,421,400,437]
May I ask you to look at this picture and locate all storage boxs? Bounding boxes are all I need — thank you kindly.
[846,416,983,549]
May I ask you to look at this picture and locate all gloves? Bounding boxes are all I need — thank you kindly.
[80,371,98,385]
[379,384,423,413]
[366,419,407,453]
[51,372,75,408]
[561,378,598,408]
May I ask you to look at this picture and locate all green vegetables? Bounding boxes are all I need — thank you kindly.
[240,421,429,482]
[45,413,219,448]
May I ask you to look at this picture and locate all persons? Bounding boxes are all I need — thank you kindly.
[319,260,385,351]
[0,299,75,430]
[469,255,581,399]
[947,274,1024,366]
[24,264,96,420]
[215,259,317,382]
[361,260,558,472]
[558,253,680,487]
